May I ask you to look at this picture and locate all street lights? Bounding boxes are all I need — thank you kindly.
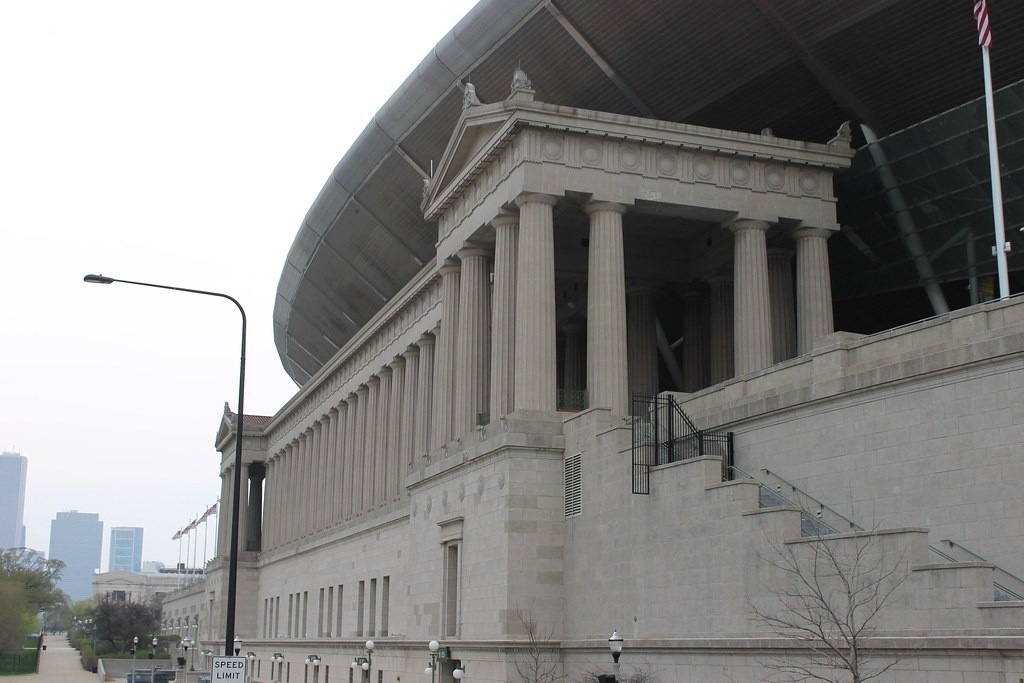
[83,274,247,657]
[75,623,78,628]
[93,623,97,660]
[151,637,158,683]
[74,617,76,628]
[429,640,440,683]
[132,636,139,683]
[88,618,93,640]
[234,635,243,657]
[609,629,625,683]
[183,637,191,683]
[84,619,88,638]
[181,641,184,658]
[189,641,194,671]
[365,640,375,683]
[78,620,82,636]
